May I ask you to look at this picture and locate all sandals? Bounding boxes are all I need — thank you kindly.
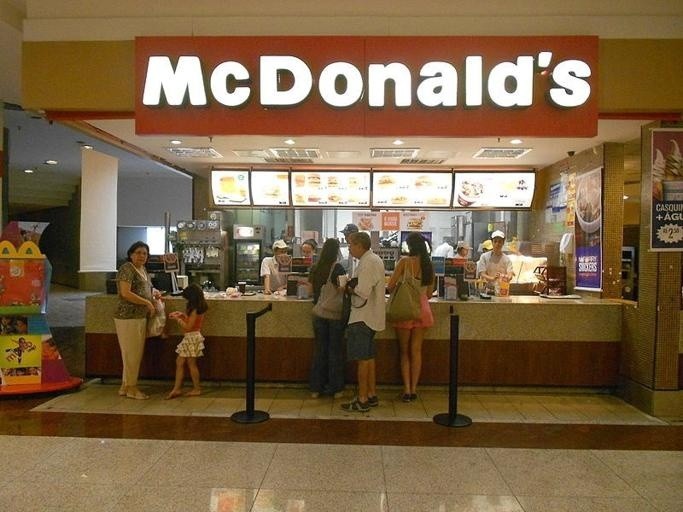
[369,395,378,406]
[340,397,369,412]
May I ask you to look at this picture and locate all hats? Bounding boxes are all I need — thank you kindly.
[340,224,358,233]
[457,241,473,249]
[482,240,493,249]
[272,240,289,250]
[491,230,505,238]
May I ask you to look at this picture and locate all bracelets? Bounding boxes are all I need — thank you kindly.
[176,318,182,326]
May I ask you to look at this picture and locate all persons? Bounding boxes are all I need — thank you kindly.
[259,232,474,411]
[475,229,517,296]
[339,223,361,272]
[481,240,494,253]
[111,241,162,400]
[164,282,207,400]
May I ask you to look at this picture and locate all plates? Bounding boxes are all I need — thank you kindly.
[405,225,422,230]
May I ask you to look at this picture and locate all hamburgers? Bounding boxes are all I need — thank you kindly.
[308,174,321,186]
[415,175,431,188]
[328,176,338,187]
[263,183,279,197]
[296,192,305,201]
[308,193,323,202]
[295,175,305,186]
[328,194,341,201]
[406,218,423,229]
[377,176,393,186]
[392,195,408,204]
[348,177,359,187]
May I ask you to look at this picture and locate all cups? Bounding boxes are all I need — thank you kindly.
[237,281,246,294]
[457,187,477,207]
[659,180,682,200]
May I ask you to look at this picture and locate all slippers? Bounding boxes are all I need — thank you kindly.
[119,390,126,396]
[166,392,182,399]
[127,391,149,400]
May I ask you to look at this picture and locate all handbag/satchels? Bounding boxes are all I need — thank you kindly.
[385,256,423,322]
[332,277,367,330]
[145,298,167,337]
[312,262,345,320]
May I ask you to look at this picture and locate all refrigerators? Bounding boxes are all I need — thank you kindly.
[231,223,264,285]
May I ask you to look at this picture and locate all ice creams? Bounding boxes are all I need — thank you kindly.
[458,177,486,206]
[665,140,682,180]
[653,148,666,202]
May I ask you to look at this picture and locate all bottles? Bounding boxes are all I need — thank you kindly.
[494,276,500,297]
[501,275,510,297]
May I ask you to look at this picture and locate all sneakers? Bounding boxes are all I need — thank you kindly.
[311,391,320,399]
[334,389,355,399]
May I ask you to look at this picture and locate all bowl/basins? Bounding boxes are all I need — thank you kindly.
[575,181,600,233]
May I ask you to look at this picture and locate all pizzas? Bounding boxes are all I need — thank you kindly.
[578,174,600,222]
